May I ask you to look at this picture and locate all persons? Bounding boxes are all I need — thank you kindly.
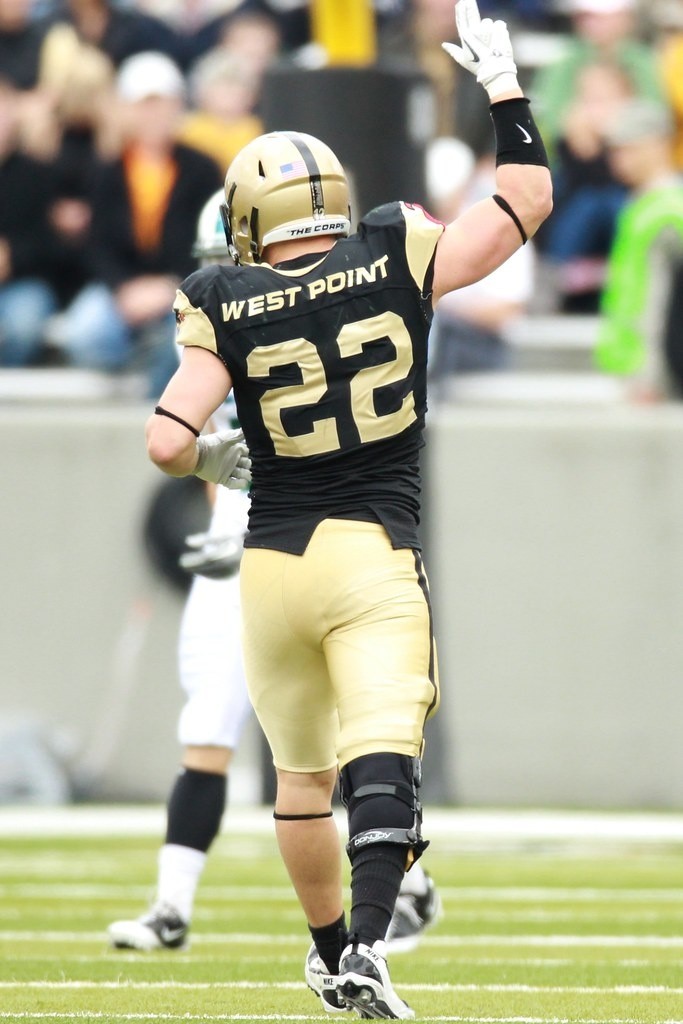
[0,0,680,392]
[146,3,556,1024]
[104,184,437,954]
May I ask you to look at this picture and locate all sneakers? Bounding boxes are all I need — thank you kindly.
[382,874,444,953]
[334,941,415,1021]
[303,941,350,1013]
[105,908,189,952]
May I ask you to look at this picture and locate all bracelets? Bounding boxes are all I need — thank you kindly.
[154,404,203,441]
[492,194,530,246]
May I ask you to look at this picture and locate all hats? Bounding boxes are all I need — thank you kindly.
[648,3,683,28]
[601,98,674,149]
[555,0,629,15]
[116,49,188,104]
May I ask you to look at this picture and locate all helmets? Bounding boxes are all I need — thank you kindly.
[219,130,352,265]
[190,190,233,258]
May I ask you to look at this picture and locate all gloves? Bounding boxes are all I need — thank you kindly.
[441,0,517,84]
[191,427,252,489]
[177,492,250,579]
